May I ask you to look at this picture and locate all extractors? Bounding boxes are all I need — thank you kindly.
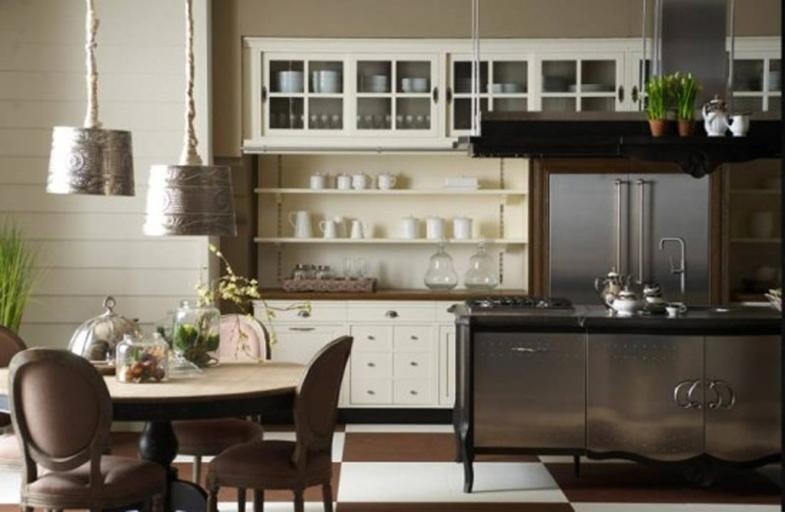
[470,1,784,179]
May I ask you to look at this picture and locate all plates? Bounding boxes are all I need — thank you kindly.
[278,71,427,93]
[487,82,611,93]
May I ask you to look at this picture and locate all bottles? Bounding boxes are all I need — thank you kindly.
[423,244,458,290]
[464,244,499,289]
[116,332,169,383]
[292,264,335,280]
[172,299,221,370]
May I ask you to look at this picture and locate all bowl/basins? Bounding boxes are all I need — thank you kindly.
[761,70,783,91]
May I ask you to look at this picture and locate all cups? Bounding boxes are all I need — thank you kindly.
[453,218,473,239]
[341,257,352,280]
[401,213,419,239]
[354,258,367,281]
[351,221,364,239]
[310,172,397,190]
[723,114,750,136]
[334,216,347,238]
[270,112,430,129]
[426,213,445,239]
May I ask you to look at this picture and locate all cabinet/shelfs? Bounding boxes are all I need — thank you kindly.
[248,152,537,295]
[712,155,785,306]
[243,38,453,147]
[440,301,586,493]
[253,297,461,413]
[722,39,785,114]
[586,324,783,467]
[445,36,652,139]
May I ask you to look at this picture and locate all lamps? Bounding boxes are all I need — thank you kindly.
[141,0,239,241]
[43,0,138,201]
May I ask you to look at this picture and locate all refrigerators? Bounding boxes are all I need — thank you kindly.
[548,173,712,307]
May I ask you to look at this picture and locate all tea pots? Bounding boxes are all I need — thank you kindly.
[592,270,633,307]
[319,220,343,239]
[702,93,730,138]
[605,285,646,318]
[288,211,319,239]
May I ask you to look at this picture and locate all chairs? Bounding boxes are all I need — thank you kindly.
[0,324,29,436]
[173,312,273,512]
[203,334,354,512]
[6,344,169,511]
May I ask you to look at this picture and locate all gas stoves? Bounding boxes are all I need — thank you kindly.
[466,294,576,318]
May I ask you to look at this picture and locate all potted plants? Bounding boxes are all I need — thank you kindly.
[669,70,704,137]
[644,76,668,136]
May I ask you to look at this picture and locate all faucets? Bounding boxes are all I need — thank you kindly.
[659,236,688,292]
